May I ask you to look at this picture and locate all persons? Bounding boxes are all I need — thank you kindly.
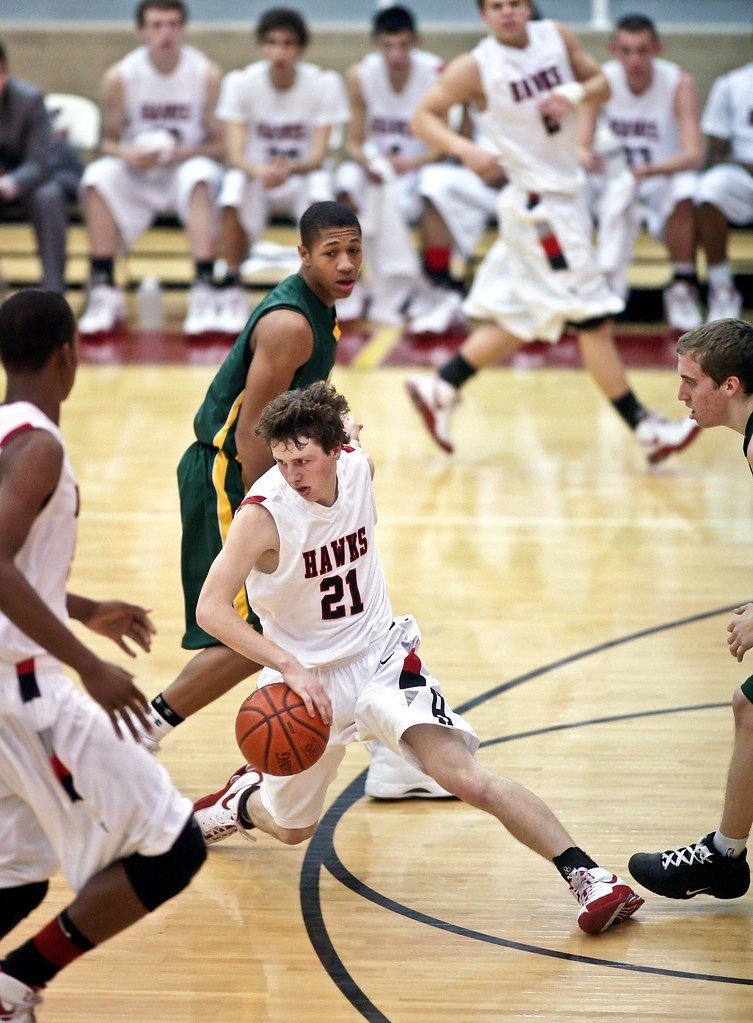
[214,0,706,340]
[191,378,646,937]
[696,0,753,324]
[402,0,704,467]
[0,40,88,298]
[76,1,224,338]
[113,199,364,763]
[0,285,212,1022]
[626,316,753,904]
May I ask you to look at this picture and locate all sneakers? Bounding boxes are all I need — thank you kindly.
[363,741,456,797]
[568,866,645,933]
[628,832,749,899]
[0,972,42,1023]
[193,763,264,844]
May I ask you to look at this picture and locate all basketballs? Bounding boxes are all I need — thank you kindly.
[234,682,331,777]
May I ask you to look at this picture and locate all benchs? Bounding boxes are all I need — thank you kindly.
[0,198,752,295]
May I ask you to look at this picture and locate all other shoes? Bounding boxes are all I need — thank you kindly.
[219,290,247,333]
[635,416,700,463]
[707,290,740,322]
[663,283,701,331]
[185,289,219,333]
[78,287,121,336]
[406,376,456,453]
